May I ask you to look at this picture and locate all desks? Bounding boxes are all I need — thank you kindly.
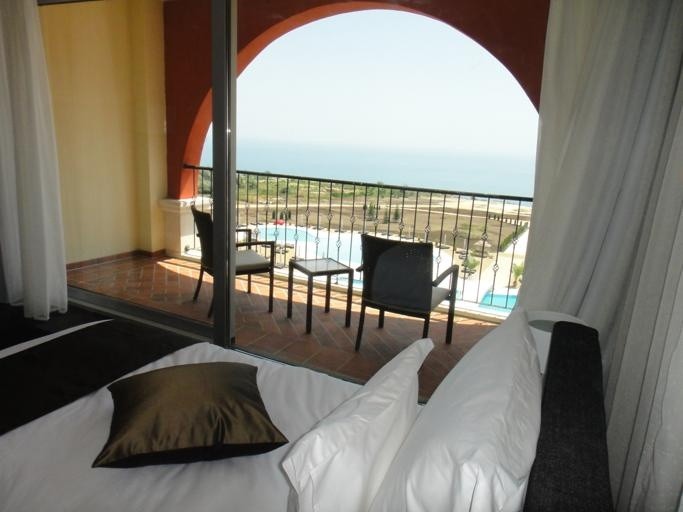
[288,258,355,334]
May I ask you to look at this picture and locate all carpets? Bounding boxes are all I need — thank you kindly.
[67,283,217,341]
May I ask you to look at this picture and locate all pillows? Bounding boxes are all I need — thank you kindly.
[281,338,433,510]
[90,358,287,467]
[370,310,543,512]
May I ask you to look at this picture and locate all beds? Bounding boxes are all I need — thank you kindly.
[0,301,617,512]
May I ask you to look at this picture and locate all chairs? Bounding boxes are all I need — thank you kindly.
[355,232,459,349]
[186,203,273,320]
